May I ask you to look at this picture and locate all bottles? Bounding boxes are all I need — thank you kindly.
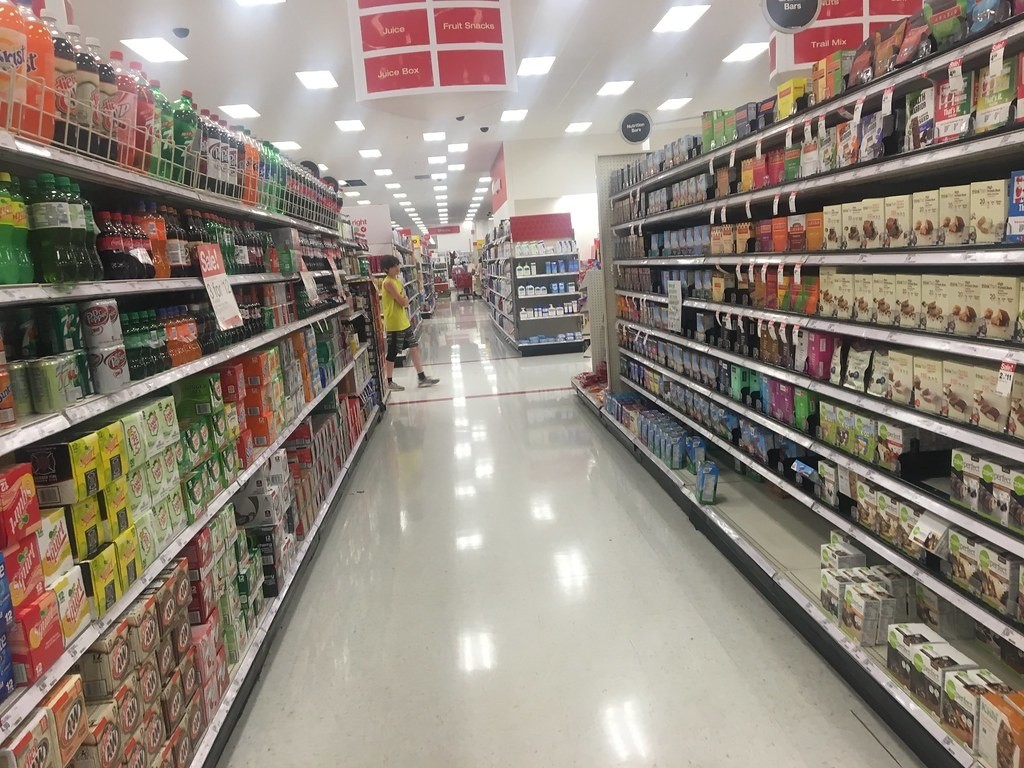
[121,275,349,381]
[346,233,369,277]
[514,239,538,256]
[515,260,532,275]
[346,324,361,356]
[0,173,343,286]
[0,1,344,230]
[516,283,535,296]
[343,284,366,318]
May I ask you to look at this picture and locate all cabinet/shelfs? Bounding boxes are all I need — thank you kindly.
[480,0,1024,768]
[0,13,448,768]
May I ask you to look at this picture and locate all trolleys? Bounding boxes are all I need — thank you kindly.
[452,266,476,302]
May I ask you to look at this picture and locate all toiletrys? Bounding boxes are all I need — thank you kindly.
[515,240,583,347]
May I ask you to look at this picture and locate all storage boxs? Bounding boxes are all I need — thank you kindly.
[0,50,1024,768]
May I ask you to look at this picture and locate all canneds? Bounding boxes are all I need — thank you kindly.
[0,298,132,428]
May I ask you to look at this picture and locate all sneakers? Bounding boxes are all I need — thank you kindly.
[387,380,404,392]
[419,376,441,387]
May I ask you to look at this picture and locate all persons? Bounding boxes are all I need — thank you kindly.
[462,261,469,272]
[381,254,440,391]
[476,256,483,299]
